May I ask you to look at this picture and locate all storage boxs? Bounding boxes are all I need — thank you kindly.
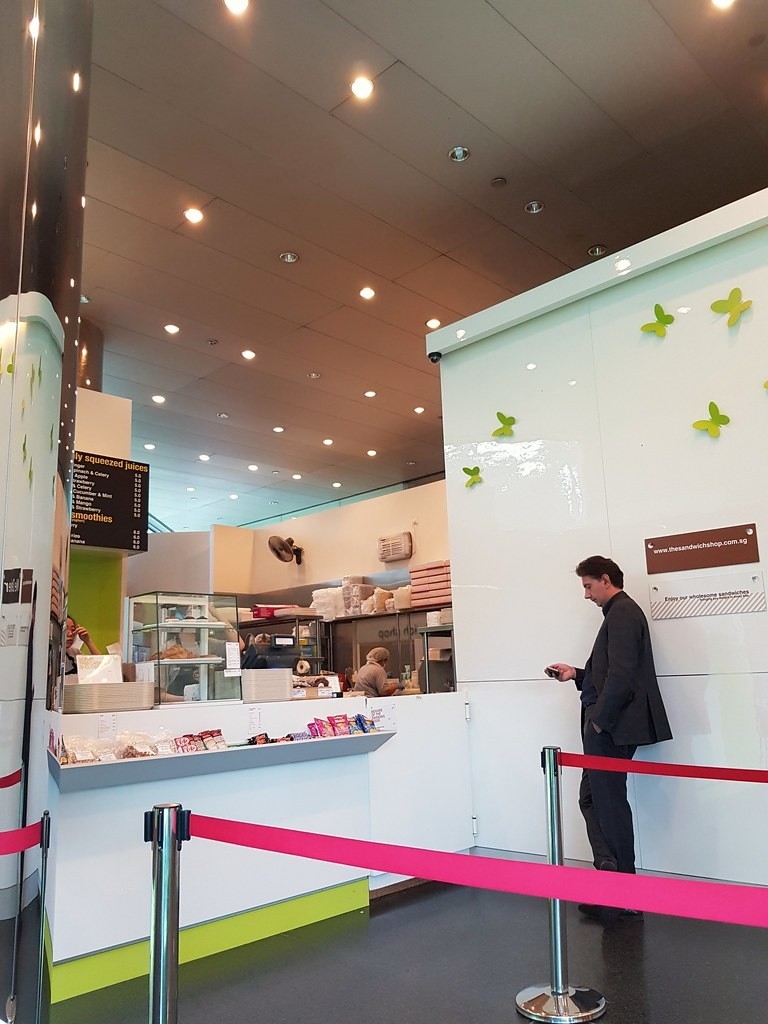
[251,604,301,620]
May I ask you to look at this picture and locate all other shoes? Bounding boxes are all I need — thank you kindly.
[600,857,620,870]
[579,900,644,920]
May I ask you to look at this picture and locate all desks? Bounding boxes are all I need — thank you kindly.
[417,625,457,694]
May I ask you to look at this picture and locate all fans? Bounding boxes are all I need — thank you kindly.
[268,536,302,565]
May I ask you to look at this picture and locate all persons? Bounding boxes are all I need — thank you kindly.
[352,646,405,698]
[59,614,101,674]
[256,634,272,644]
[544,555,674,911]
[153,601,245,702]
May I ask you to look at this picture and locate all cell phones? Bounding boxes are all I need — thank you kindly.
[547,667,559,675]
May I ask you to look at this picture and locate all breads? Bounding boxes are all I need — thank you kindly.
[149,644,202,661]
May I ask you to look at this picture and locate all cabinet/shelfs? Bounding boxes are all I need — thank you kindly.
[128,591,243,709]
[240,615,324,674]
